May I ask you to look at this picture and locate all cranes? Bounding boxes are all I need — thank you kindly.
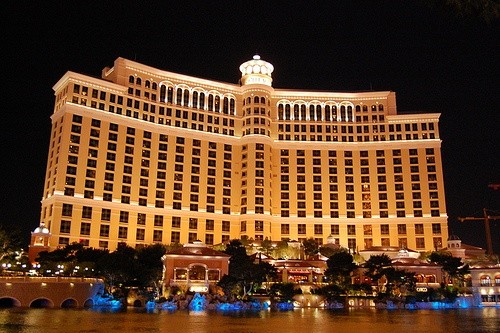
[458,208,500,258]
